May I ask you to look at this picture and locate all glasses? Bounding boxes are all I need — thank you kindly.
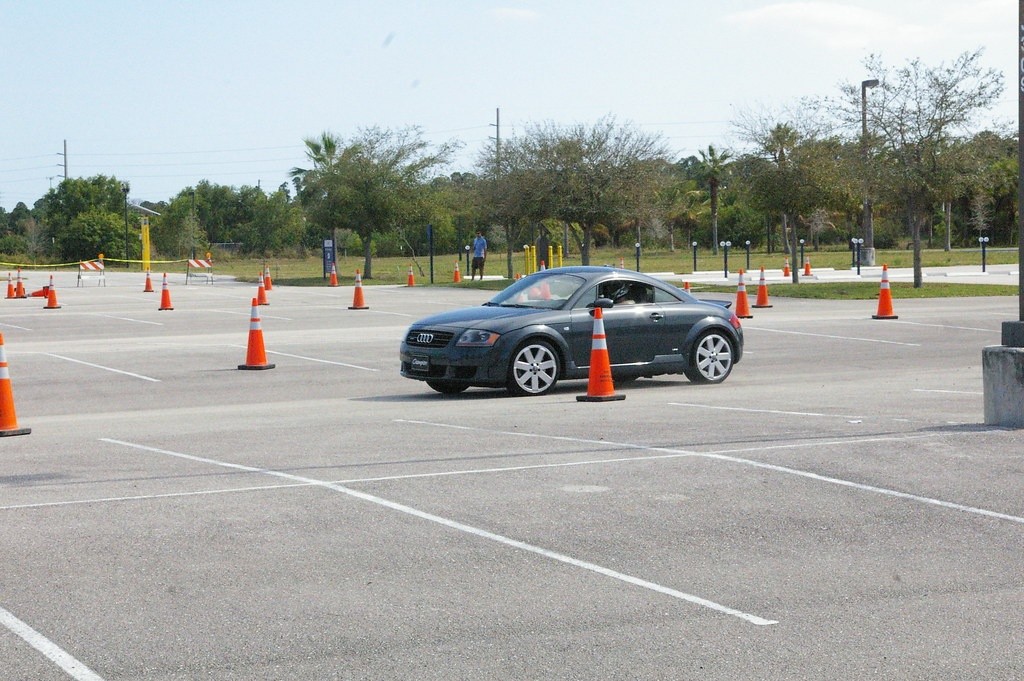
[476,233,480,234]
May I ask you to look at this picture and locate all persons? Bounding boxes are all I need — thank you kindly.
[470,229,487,280]
[602,280,640,304]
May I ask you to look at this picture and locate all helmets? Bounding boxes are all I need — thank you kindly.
[606,280,629,301]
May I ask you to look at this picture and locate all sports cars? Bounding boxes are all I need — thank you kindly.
[399,266,744,398]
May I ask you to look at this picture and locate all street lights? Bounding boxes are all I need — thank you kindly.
[187,191,196,260]
[861,79,880,248]
[122,188,130,268]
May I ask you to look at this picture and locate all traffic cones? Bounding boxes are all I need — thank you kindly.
[256,271,271,306]
[575,307,627,402]
[801,253,814,276]
[871,264,899,319]
[783,256,791,277]
[453,260,462,283]
[348,268,369,309]
[26,286,49,299]
[43,274,62,309]
[327,262,341,287]
[734,266,754,318]
[403,262,416,287]
[682,281,691,294]
[157,272,174,310]
[264,264,274,291]
[13,266,28,299]
[513,273,525,304]
[4,272,17,299]
[539,261,551,300]
[238,297,276,370]
[0,331,32,438]
[751,266,773,308]
[142,267,155,293]
